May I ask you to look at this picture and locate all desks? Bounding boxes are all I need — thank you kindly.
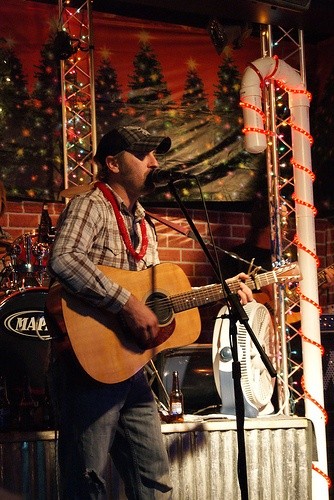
[1,416,319,500]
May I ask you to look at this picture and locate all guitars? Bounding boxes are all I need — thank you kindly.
[44,262,304,386]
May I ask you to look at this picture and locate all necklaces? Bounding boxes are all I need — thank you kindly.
[93,181,149,261]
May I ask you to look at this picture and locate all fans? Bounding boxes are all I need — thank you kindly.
[212,299,277,418]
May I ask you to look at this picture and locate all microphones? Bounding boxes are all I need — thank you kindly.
[150,169,196,182]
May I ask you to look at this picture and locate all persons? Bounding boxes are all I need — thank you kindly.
[0,180,17,274]
[46,125,253,500]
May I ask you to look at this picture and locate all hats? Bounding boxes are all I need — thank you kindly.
[96,126,171,164]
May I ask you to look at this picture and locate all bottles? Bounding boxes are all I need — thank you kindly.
[39,201,53,226]
[170,370,184,423]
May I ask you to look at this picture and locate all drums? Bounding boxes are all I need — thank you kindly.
[7,230,55,280]
[0,282,52,429]
